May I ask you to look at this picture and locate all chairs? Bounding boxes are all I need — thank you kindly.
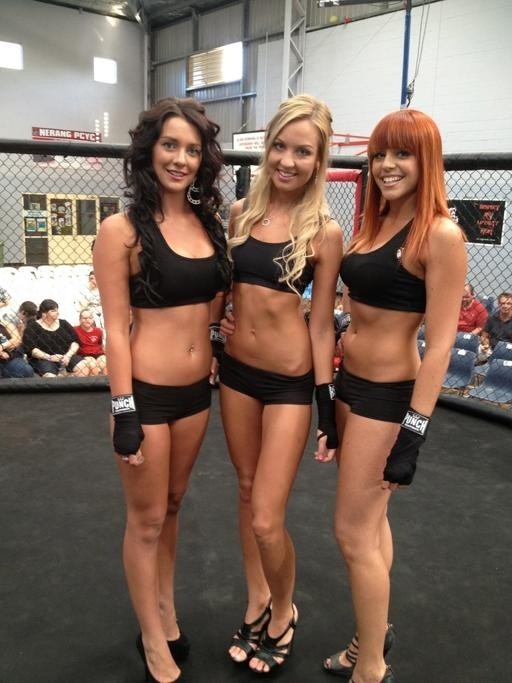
[417,324,512,407]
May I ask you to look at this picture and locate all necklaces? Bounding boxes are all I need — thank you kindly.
[262,195,301,226]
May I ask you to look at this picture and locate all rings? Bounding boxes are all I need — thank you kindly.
[121,455,129,461]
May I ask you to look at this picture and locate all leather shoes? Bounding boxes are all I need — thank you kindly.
[136,621,189,683]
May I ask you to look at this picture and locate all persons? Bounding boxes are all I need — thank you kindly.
[0,270,135,377]
[456,282,488,337]
[317,104,470,683]
[217,92,341,677]
[477,328,492,365]
[92,94,226,682]
[480,292,511,350]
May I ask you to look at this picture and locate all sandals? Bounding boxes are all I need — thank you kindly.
[324,623,397,683]
[227,593,295,677]
